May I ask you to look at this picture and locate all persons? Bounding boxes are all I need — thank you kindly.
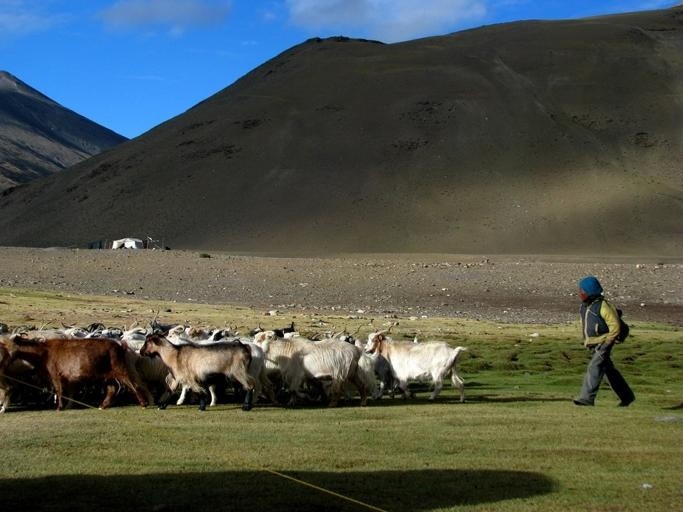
[573,276,635,407]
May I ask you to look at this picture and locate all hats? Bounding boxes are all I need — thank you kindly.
[578,277,603,296]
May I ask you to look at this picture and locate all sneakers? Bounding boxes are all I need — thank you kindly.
[573,397,635,407]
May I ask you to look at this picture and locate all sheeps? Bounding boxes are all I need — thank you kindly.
[0,309,467,412]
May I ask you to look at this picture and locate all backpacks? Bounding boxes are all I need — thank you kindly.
[613,307,630,345]
